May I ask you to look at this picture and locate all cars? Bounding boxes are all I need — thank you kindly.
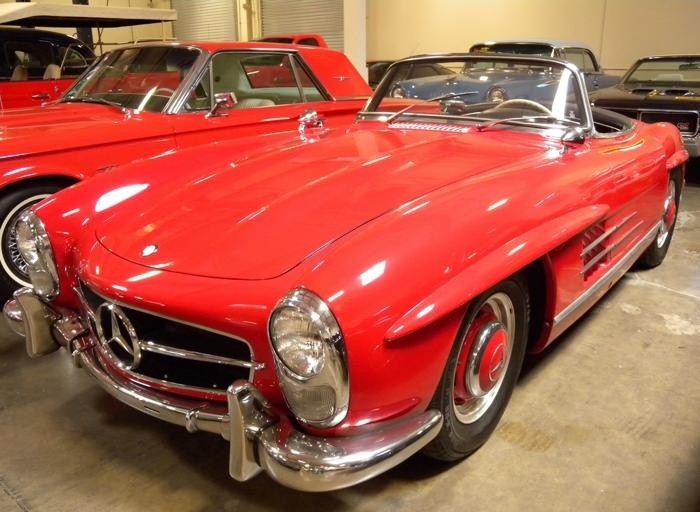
[391,39,636,105]
[369,62,456,88]
[0,27,98,109]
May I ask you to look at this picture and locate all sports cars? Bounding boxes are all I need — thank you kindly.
[588,56,699,187]
[3,55,688,495]
[0,41,437,299]
